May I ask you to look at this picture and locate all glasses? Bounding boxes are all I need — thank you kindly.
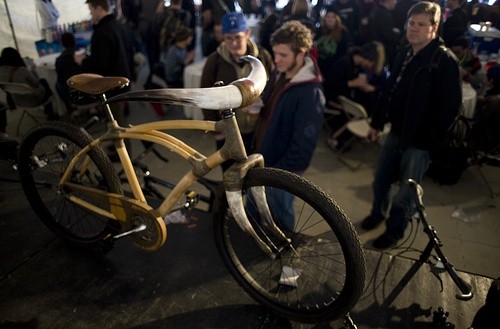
[224,36,244,43]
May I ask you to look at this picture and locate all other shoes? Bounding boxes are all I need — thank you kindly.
[337,140,352,153]
[373,234,403,249]
[362,210,384,230]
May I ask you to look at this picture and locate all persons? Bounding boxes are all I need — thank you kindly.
[360,2,463,248]
[0,0,500,188]
[255,21,326,253]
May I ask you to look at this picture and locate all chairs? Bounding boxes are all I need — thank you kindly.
[336,95,383,170]
[0,66,58,137]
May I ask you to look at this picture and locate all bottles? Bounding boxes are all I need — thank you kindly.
[40,18,90,43]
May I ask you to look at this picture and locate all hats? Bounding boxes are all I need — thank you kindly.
[176,25,191,41]
[222,12,248,34]
[359,43,376,58]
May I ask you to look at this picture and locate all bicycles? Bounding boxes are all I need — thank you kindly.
[340,179,476,329]
[15,53,368,323]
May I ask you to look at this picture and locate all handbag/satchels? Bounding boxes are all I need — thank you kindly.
[9,66,52,108]
